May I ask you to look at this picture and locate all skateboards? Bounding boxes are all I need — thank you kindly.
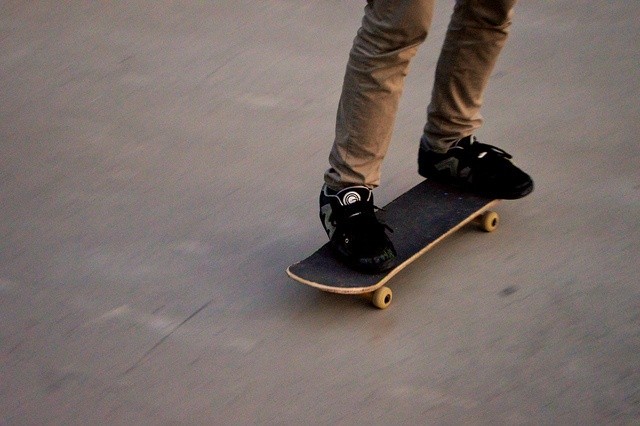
[286,178,502,309]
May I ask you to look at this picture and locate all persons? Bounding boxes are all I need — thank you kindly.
[320,1,534,274]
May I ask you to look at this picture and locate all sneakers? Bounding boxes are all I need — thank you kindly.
[418,132,534,199]
[319,181,397,273]
